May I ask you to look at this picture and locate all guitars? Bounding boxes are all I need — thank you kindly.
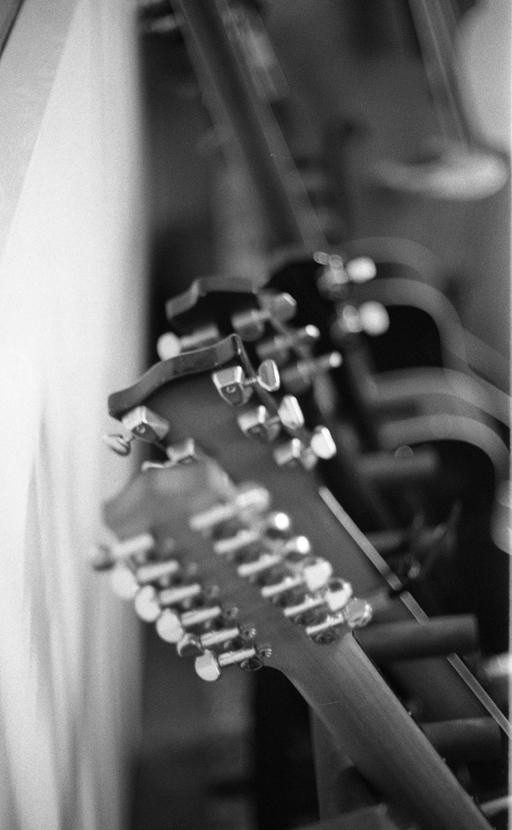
[152,276,509,701]
[257,239,510,574]
[90,460,493,830]
[138,3,510,406]
[98,333,510,830]
